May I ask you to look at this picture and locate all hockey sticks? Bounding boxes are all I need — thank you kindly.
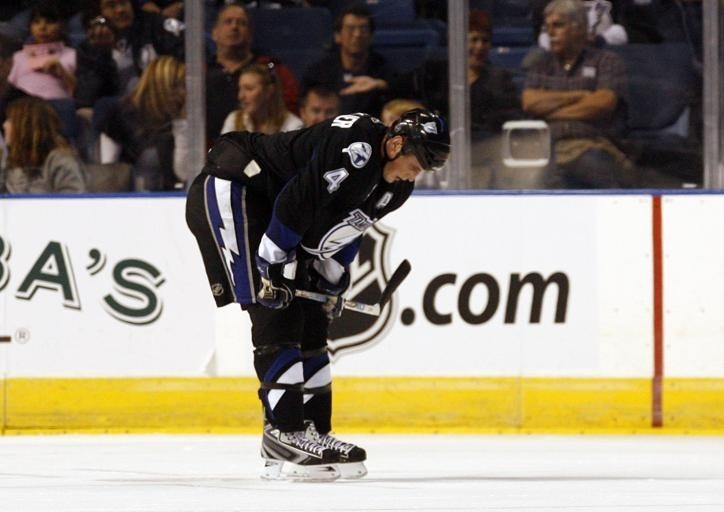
[258,260,411,316]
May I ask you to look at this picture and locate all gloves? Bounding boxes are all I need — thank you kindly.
[302,259,350,321]
[252,250,293,311]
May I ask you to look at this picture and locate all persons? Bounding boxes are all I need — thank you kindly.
[186,109,453,466]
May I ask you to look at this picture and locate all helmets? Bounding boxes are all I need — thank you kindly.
[389,108,450,169]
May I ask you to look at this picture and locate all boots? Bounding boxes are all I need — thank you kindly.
[301,419,368,464]
[259,414,338,466]
